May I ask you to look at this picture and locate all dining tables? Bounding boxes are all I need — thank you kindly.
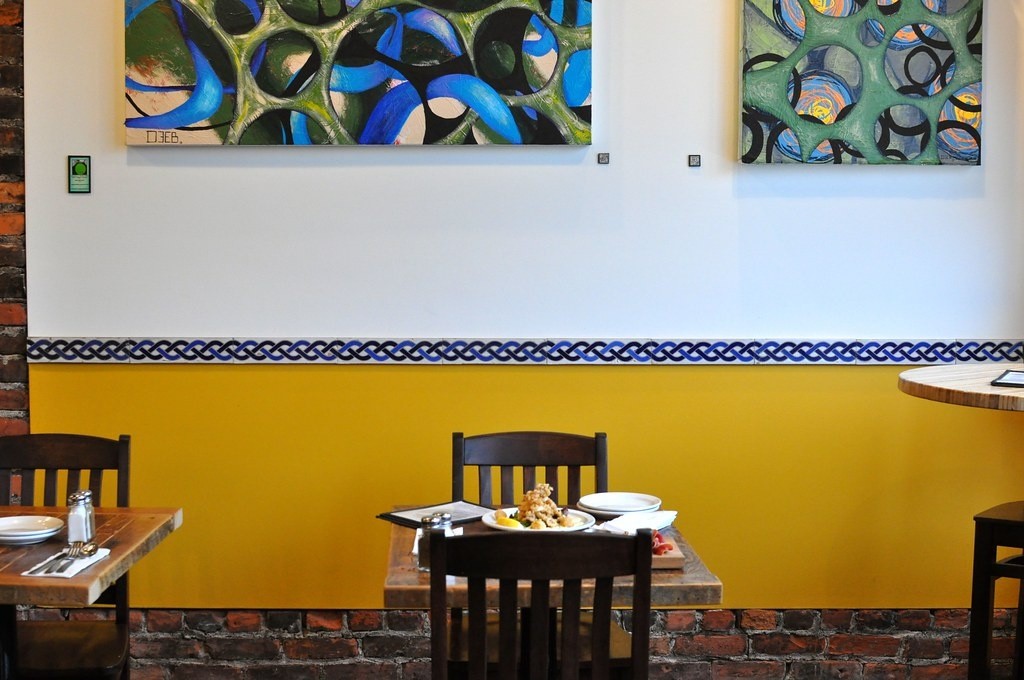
[382,501,722,678]
[0,505,183,680]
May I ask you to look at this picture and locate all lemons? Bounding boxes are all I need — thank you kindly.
[496,517,522,528]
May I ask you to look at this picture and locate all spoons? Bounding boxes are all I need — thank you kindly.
[56,541,98,573]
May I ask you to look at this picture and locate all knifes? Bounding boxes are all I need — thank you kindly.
[29,553,67,574]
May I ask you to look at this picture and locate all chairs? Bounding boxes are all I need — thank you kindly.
[967,501,1024,680]
[0,434,131,680]
[429,433,654,680]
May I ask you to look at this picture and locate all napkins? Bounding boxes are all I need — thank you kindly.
[595,511,678,537]
[21,548,111,578]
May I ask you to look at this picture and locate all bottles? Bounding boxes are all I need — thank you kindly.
[416,513,452,573]
[67,489,97,545]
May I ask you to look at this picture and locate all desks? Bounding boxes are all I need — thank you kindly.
[897,363,1024,412]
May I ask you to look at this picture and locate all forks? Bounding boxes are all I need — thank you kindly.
[46,541,84,573]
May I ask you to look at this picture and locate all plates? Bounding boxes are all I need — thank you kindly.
[576,491,661,520]
[0,515,66,544]
[482,507,596,531]
